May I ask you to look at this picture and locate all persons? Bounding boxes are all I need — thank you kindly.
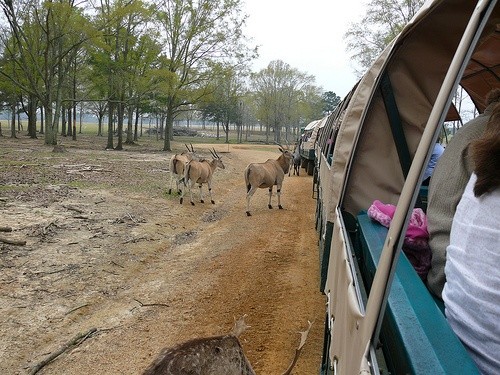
[423,87,500,319]
[422,130,444,189]
[439,103,500,374]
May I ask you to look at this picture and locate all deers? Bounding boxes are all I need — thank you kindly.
[180,147,226,206]
[167,142,198,196]
[289,138,302,176]
[245,138,295,216]
